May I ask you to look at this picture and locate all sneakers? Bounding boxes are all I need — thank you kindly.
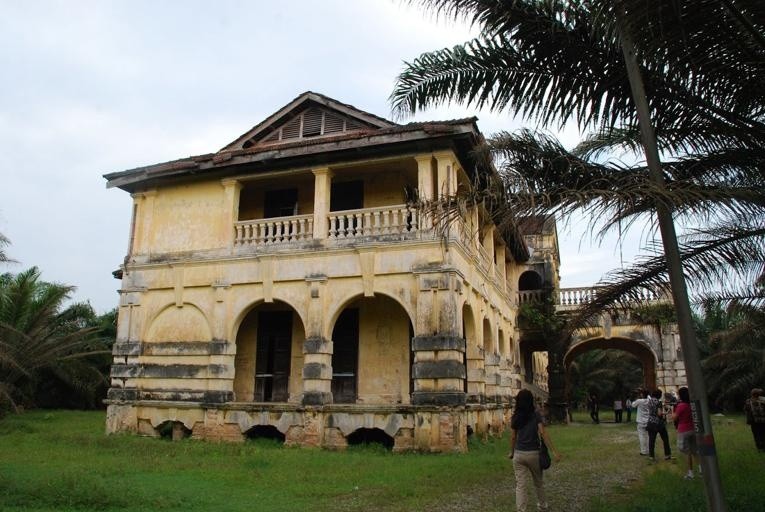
[684,475,695,481]
[664,455,673,462]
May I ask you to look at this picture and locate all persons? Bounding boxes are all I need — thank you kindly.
[507,388,561,511]
[743,388,765,449]
[612,386,704,481]
[587,393,600,424]
[759,388,765,401]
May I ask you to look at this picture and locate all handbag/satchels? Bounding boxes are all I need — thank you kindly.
[646,422,658,432]
[539,436,551,470]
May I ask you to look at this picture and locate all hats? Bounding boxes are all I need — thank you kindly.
[752,389,762,395]
